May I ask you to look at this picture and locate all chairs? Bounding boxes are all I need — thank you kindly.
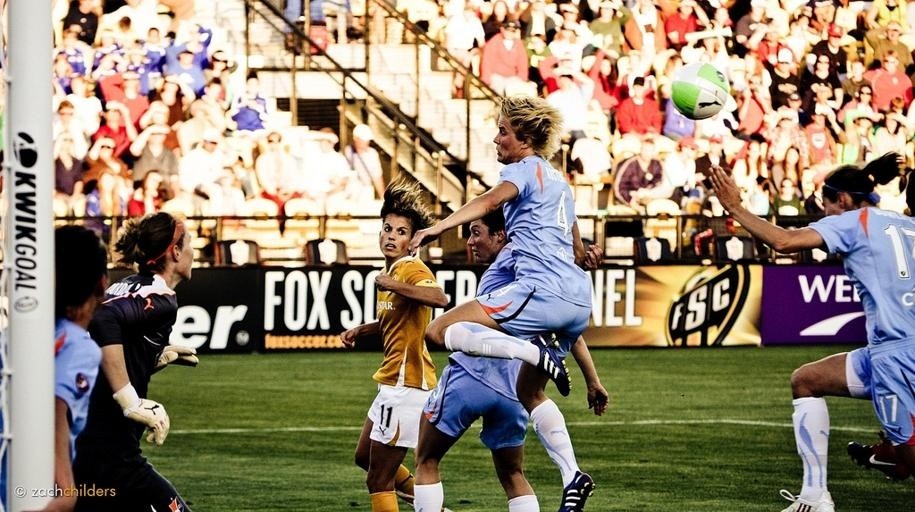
[1,0,914,271]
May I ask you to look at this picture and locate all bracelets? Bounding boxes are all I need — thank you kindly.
[112,382,139,414]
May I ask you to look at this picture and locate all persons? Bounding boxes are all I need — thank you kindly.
[414,208,608,512]
[848,430,911,482]
[1,226,108,510]
[405,94,603,512]
[73,213,200,511]
[339,178,451,511]
[50,0,915,240]
[709,151,915,512]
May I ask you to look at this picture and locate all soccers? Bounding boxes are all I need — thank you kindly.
[671,61,728,120]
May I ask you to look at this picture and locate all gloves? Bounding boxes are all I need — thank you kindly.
[113,382,169,447]
[153,346,199,369]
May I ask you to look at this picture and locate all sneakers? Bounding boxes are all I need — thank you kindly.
[780,493,835,512]
[848,438,911,481]
[533,331,570,397]
[559,472,595,512]
